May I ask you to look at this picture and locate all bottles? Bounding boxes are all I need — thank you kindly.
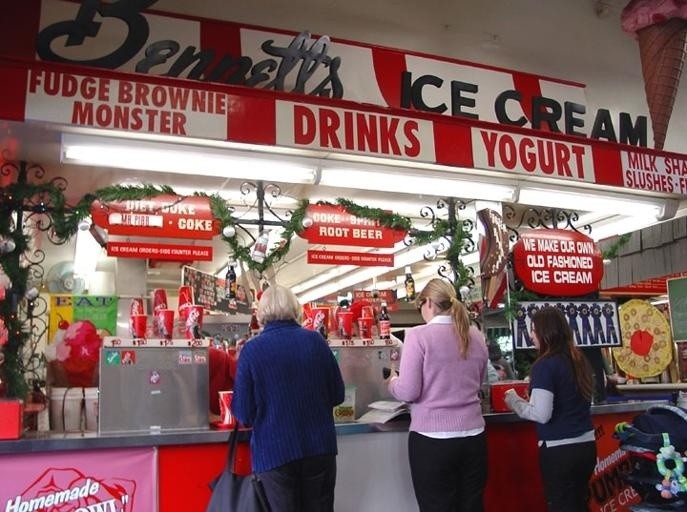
[376,302,391,340]
[402,266,416,303]
[224,257,237,301]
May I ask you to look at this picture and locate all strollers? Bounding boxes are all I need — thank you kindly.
[612,404,687,512]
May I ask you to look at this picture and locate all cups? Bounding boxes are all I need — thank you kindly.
[331,384,358,425]
[337,312,353,341]
[129,285,203,340]
[358,316,373,340]
[249,237,268,264]
[51,385,97,431]
[309,307,330,343]
[218,392,236,425]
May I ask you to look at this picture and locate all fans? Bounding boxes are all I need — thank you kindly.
[45,260,85,294]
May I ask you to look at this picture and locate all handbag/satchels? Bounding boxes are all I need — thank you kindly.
[204,472,268,512]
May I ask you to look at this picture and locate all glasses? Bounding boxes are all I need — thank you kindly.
[418,300,426,312]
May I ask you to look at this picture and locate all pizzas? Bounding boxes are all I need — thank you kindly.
[611,298,673,379]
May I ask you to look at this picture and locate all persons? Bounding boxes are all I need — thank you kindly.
[504,305,597,512]
[382,278,490,512]
[231,285,344,512]
[209,347,240,422]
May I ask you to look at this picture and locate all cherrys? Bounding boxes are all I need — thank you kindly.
[56,313,69,329]
